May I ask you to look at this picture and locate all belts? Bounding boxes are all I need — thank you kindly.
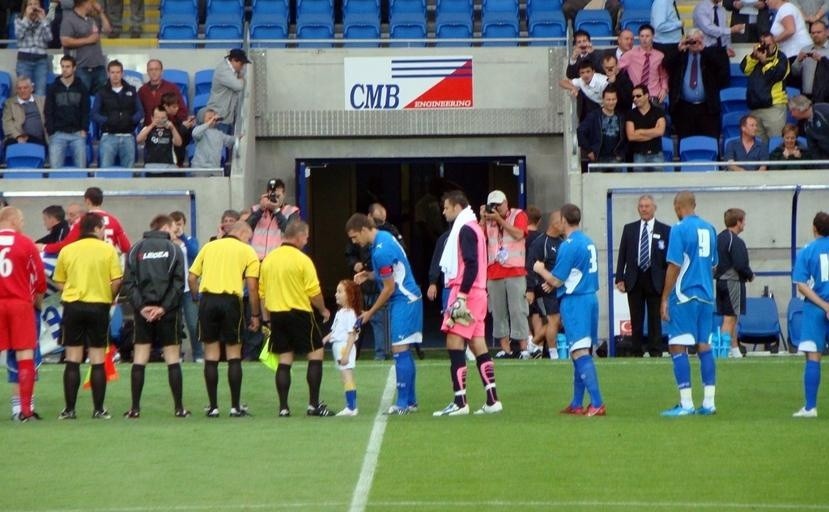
[679,97,707,108]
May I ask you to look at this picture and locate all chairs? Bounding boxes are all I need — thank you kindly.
[734,297,779,351]
[1,14,18,50]
[0,63,229,180]
[612,62,811,172]
[157,0,655,50]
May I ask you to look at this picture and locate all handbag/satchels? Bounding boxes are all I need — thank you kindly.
[596,334,645,358]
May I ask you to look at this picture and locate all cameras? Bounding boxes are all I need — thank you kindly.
[161,118,171,126]
[485,204,496,214]
[267,179,279,204]
[579,45,587,49]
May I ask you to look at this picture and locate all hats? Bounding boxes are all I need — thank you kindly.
[486,190,507,205]
[227,48,252,64]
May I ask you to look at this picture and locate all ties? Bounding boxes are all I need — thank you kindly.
[640,53,651,87]
[712,5,722,52]
[673,0,686,38]
[689,52,697,90]
[639,222,650,272]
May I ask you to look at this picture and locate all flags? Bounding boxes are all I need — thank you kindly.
[83,343,120,388]
[259,336,280,371]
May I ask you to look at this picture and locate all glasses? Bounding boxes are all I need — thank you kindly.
[631,94,645,99]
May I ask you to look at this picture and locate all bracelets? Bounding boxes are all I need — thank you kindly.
[249,310,263,319]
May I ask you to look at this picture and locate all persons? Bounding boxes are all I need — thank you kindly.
[789,211,828,418]
[659,190,721,419]
[321,278,366,416]
[7,288,42,421]
[189,220,263,418]
[0,0,250,176]
[255,220,336,417]
[125,212,192,419]
[432,191,503,417]
[0,205,48,427]
[342,212,424,416]
[53,213,114,422]
[533,204,608,416]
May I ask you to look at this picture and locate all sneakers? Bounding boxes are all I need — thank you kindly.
[122,408,141,421]
[175,407,192,419]
[432,400,503,417]
[56,407,76,420]
[728,349,743,358]
[494,343,559,360]
[11,411,43,425]
[279,403,360,417]
[659,401,717,417]
[204,404,253,418]
[382,403,420,417]
[559,403,608,417]
[791,405,818,418]
[92,407,113,420]
